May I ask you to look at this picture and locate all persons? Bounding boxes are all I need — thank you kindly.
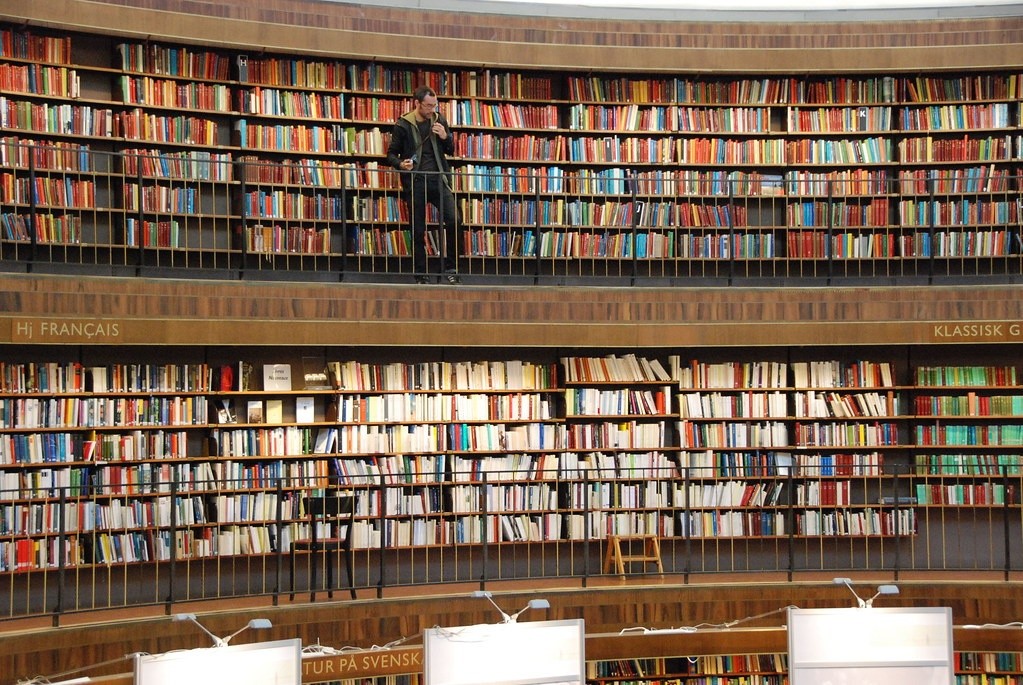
[387,85,463,286]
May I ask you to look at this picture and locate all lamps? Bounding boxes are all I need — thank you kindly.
[172,613,229,648]
[471,590,516,624]
[210,619,272,648]
[865,585,899,608]
[832,578,865,608]
[497,599,550,624]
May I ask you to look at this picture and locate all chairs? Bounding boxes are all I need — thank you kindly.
[289,495,359,603]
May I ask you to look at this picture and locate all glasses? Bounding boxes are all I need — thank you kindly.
[419,101,436,109]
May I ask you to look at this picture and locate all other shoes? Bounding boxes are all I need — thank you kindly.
[444,276,463,285]
[414,275,431,284]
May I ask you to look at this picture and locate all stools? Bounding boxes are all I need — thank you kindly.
[604,533,665,581]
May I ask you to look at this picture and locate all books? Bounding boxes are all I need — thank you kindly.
[0,352,1023,575]
[0,21,1021,258]
[319,649,1023,685]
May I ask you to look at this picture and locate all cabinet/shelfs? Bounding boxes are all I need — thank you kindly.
[1,0,1022,685]
